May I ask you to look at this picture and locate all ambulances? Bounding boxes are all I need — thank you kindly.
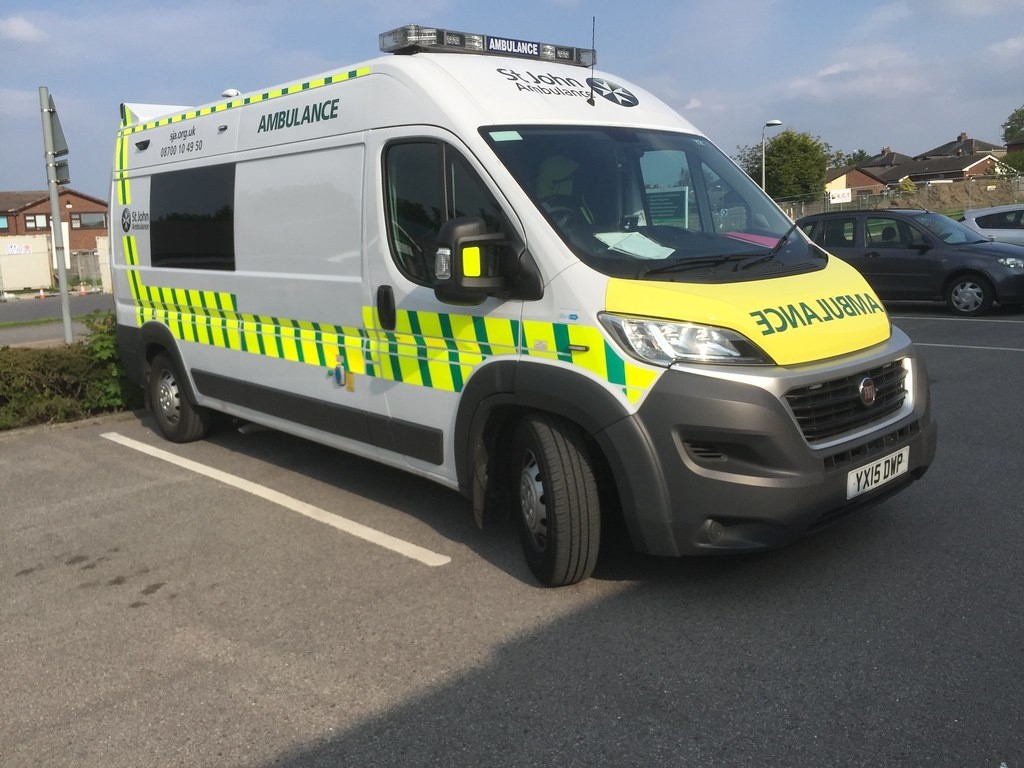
[109,24,938,587]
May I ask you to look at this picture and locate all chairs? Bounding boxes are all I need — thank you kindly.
[882,228,897,241]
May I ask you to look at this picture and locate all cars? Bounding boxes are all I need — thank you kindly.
[794,210,1024,316]
[939,204,1024,245]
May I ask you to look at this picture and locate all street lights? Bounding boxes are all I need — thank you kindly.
[762,120,782,191]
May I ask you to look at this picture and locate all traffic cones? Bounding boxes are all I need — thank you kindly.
[80,282,86,295]
[39,287,44,300]
